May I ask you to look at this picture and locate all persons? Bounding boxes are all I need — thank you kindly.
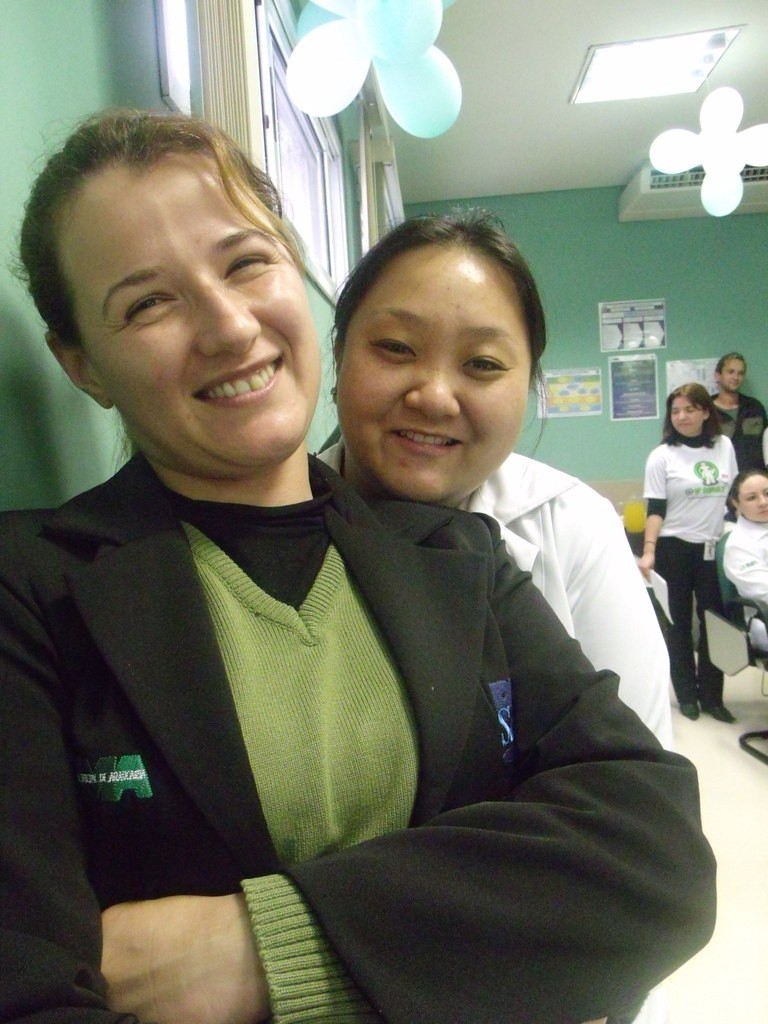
[709,352,768,473]
[0,110,716,1024]
[722,468,768,653]
[637,383,741,723]
[317,205,674,754]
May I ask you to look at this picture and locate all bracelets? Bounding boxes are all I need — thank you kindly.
[644,541,657,545]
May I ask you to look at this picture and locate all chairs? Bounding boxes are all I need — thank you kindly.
[704,528,768,765]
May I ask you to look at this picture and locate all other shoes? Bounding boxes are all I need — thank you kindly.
[702,701,736,723]
[681,701,699,720]
